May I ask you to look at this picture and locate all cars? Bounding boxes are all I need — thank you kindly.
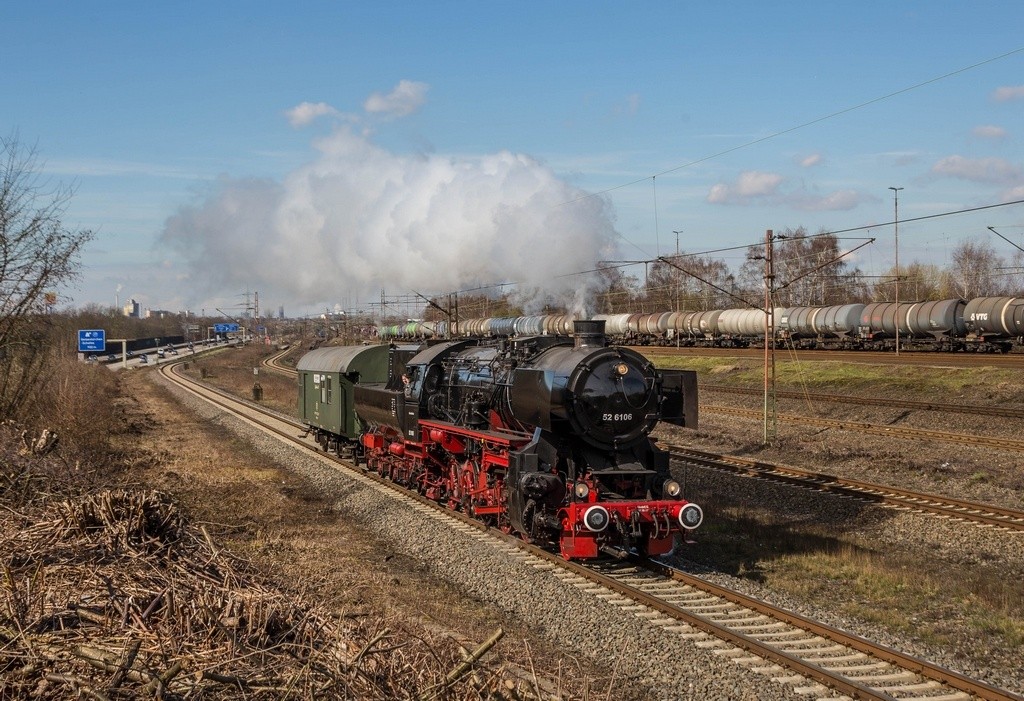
[203,339,228,346]
[173,351,178,355]
[88,354,97,360]
[127,351,133,355]
[109,355,115,360]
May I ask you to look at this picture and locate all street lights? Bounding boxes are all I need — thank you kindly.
[672,231,683,350]
[890,187,904,356]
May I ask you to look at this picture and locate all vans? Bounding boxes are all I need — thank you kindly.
[158,348,164,357]
[138,354,147,363]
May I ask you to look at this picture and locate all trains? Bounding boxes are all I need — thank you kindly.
[375,295,1024,355]
[297,302,705,562]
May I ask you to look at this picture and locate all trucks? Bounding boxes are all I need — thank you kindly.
[168,346,173,352]
[187,345,193,351]
